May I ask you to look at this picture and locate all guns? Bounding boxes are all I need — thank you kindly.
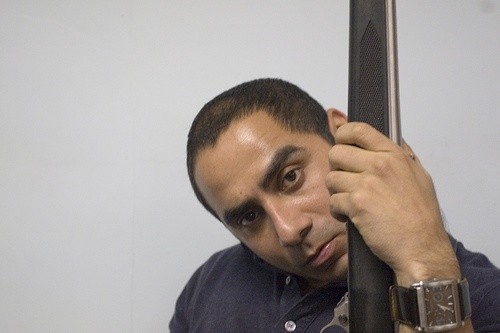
[316,0,405,333]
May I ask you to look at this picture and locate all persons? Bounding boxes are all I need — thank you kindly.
[169,77,499,333]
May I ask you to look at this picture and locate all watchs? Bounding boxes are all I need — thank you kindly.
[388,278,472,333]
